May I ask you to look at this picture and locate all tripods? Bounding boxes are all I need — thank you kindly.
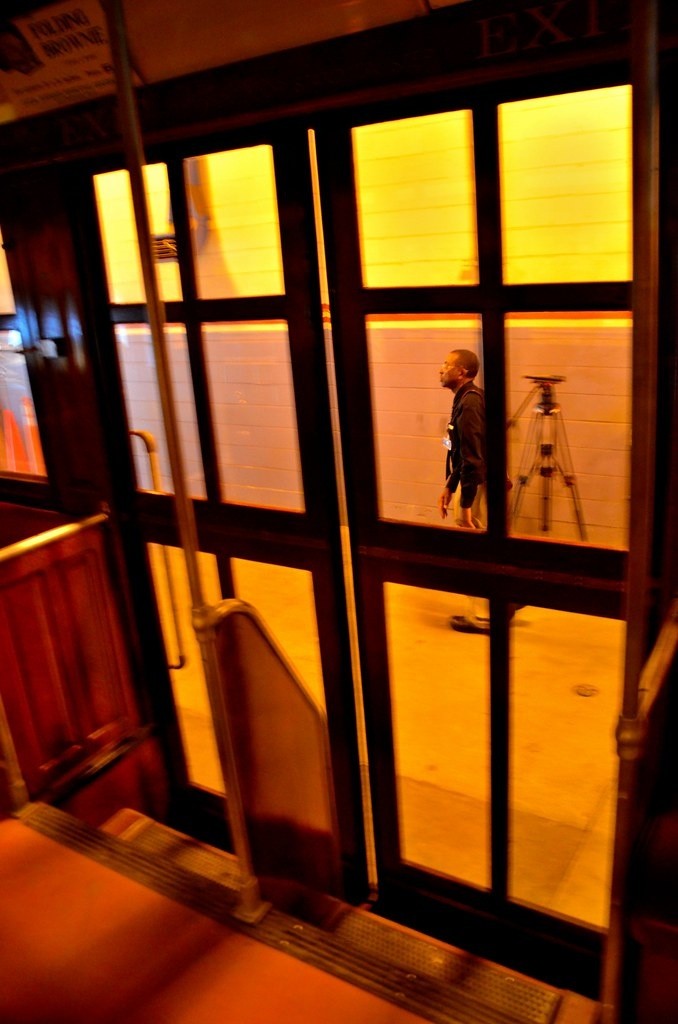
[508,405,588,540]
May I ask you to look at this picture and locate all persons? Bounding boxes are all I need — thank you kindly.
[440,349,490,635]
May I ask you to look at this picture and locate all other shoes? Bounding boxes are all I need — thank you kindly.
[450,613,492,636]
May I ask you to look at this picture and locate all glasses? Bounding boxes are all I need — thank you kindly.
[442,363,462,372]
